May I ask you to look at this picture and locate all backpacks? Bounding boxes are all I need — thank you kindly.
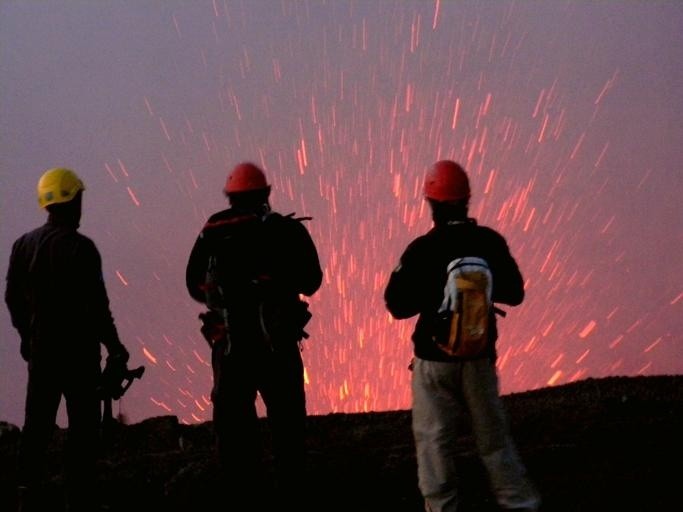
[424,226,492,358]
[200,233,274,360]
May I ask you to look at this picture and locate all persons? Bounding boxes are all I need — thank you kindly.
[1,166,132,490]
[183,161,326,501]
[381,158,547,512]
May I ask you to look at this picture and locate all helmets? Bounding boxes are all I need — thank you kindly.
[37,168,83,208]
[424,160,471,202]
[225,163,267,194]
